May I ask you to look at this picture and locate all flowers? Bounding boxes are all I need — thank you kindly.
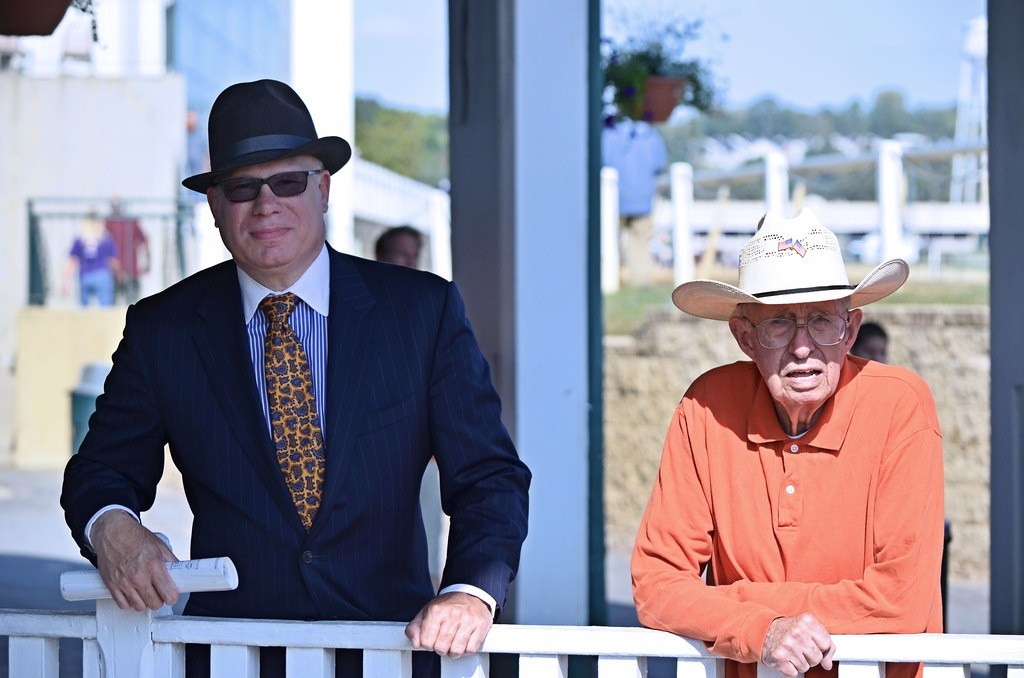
[598,3,730,143]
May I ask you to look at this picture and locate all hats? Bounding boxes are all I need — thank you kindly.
[182,79,352,194]
[672,207,909,321]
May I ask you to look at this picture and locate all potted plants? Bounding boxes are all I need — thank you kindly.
[0,0,99,43]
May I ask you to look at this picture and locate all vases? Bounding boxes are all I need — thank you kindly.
[612,74,689,126]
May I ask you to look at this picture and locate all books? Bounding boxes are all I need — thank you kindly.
[62,553,240,601]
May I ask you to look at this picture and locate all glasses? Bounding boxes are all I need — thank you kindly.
[213,170,320,203]
[741,308,849,350]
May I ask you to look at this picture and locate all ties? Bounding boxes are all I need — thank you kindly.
[257,292,327,532]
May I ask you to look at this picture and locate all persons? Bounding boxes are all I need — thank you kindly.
[850,319,889,365]
[99,195,153,304]
[631,209,945,678]
[59,79,534,678]
[60,203,129,306]
[373,225,424,268]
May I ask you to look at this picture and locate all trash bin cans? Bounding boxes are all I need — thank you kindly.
[69,365,112,455]
